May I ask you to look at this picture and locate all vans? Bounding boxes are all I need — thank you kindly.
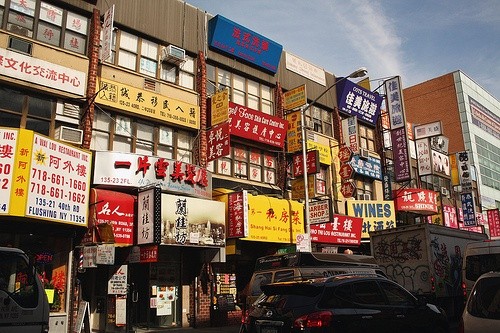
[0,247,51,333]
[459,238,500,309]
[245,253,390,315]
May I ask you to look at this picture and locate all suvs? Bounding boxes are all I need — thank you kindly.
[460,271,500,333]
[239,272,452,333]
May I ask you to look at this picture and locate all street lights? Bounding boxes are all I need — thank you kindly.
[300,68,369,249]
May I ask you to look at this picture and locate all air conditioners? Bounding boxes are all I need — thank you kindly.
[359,194,371,201]
[359,149,369,158]
[162,45,186,65]
[306,120,315,128]
[55,125,83,146]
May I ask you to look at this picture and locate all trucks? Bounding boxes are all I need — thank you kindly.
[369,222,490,312]
